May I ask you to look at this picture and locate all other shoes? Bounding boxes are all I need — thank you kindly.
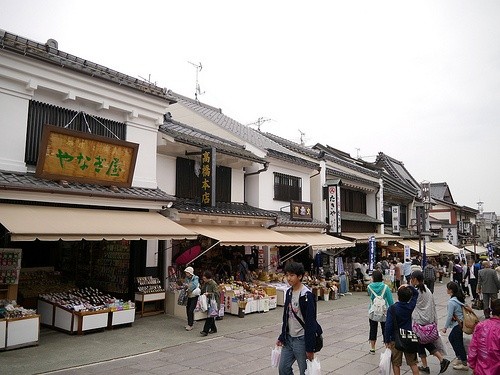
[184,324,194,331]
[453,360,462,365]
[369,349,375,355]
[418,366,430,374]
[453,364,469,371]
[209,330,217,334]
[440,359,450,373]
[200,330,208,336]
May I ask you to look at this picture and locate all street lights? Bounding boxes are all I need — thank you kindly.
[471,223,481,262]
[413,195,421,257]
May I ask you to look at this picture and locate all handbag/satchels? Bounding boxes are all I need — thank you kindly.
[177,287,196,305]
[379,349,394,375]
[431,332,448,356]
[305,358,321,375]
[198,295,208,312]
[472,292,484,310]
[271,345,283,369]
[412,321,440,344]
[313,320,323,353]
[190,275,201,295]
[209,293,219,317]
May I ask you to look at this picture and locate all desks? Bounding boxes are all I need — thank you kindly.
[134,292,166,318]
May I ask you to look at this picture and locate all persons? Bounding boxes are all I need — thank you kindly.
[181,255,500,375]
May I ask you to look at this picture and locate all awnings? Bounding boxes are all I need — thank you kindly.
[0,202,488,258]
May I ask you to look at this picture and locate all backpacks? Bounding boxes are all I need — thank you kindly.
[447,300,479,335]
[367,284,389,322]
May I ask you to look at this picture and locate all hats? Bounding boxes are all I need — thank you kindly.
[184,267,194,275]
